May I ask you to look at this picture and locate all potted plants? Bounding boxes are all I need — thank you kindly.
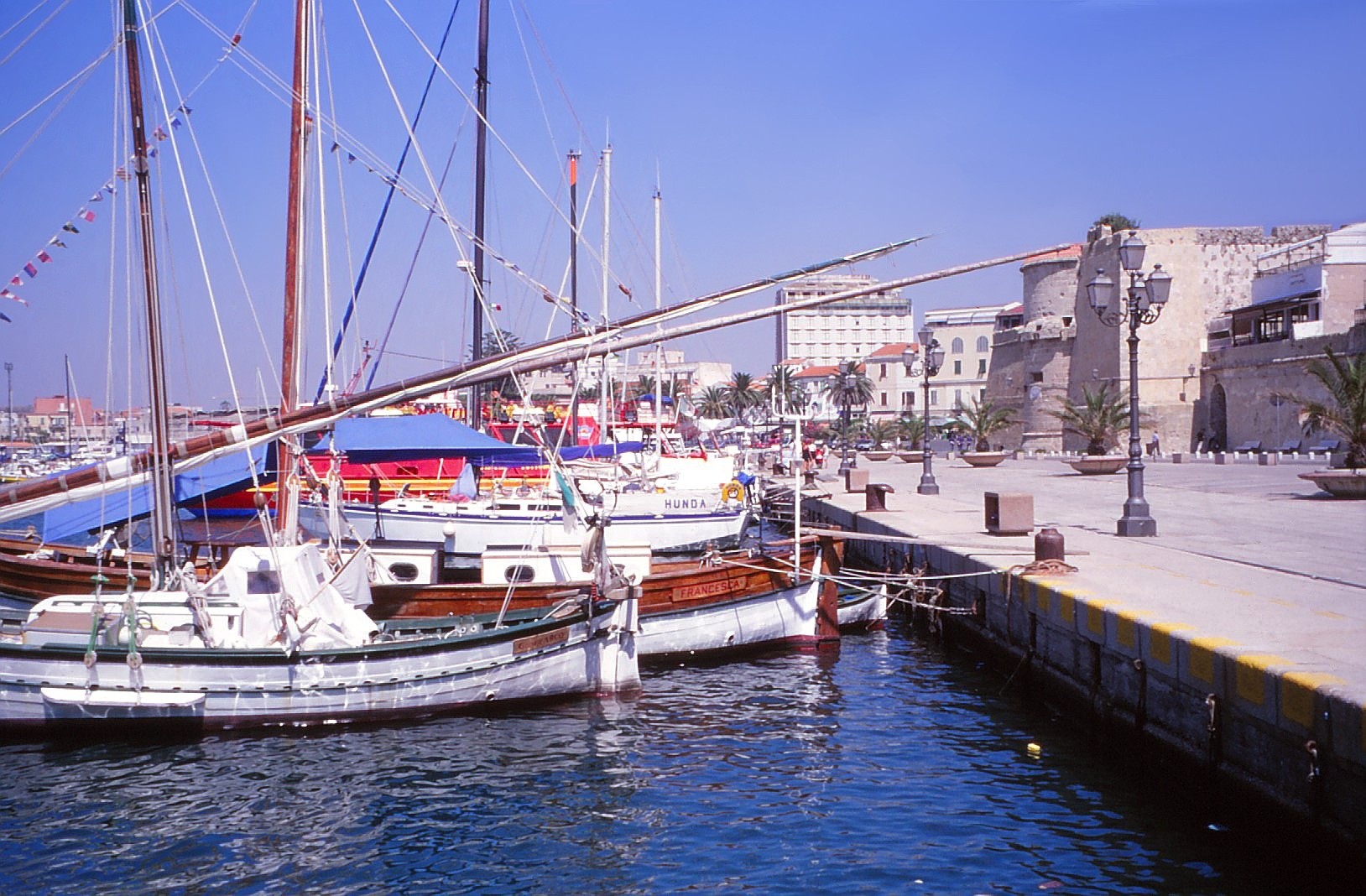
[1264,341,1366,500]
[830,415,943,463]
[1038,379,1150,475]
[959,394,1019,465]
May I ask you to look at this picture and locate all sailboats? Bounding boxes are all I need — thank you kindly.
[0,0,1074,729]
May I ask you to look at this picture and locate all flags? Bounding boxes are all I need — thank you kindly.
[0,33,594,323]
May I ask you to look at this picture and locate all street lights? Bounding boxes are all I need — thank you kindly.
[774,391,789,464]
[791,379,819,437]
[900,321,946,495]
[827,355,858,476]
[3,362,15,448]
[1083,228,1175,536]
[756,397,774,449]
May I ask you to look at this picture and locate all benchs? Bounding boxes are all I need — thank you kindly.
[1273,440,1302,454]
[67,556,74,563]
[109,561,116,567]
[1308,440,1340,452]
[1235,441,1262,454]
[395,620,524,638]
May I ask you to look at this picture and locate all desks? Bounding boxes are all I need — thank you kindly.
[178,539,261,569]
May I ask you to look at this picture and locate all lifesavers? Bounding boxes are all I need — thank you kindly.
[723,482,744,502]
[582,527,603,572]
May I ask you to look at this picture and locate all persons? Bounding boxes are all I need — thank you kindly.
[947,431,972,450]
[1150,430,1160,457]
[1196,428,1221,453]
[789,439,829,469]
